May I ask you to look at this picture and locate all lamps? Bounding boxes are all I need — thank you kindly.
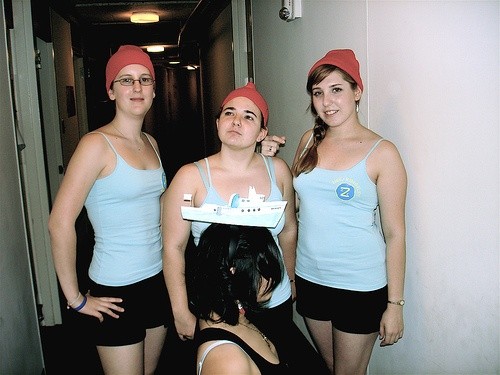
[130,13,160,23]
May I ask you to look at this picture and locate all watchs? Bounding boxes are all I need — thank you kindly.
[387,300,405,305]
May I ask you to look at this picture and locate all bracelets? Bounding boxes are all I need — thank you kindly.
[67,292,81,309]
[74,295,87,312]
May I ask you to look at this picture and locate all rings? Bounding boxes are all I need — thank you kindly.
[269,146,273,151]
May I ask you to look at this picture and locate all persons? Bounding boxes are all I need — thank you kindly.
[48,46,407,375]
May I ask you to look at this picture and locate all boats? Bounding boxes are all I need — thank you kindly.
[180,185,289,229]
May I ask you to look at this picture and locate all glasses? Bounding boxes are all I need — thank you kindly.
[113,74,154,86]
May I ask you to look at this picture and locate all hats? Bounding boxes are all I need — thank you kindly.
[220,82,268,129]
[106,45,155,93]
[308,49,364,94]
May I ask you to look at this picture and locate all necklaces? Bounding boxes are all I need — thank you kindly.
[112,123,143,152]
[237,320,271,348]
